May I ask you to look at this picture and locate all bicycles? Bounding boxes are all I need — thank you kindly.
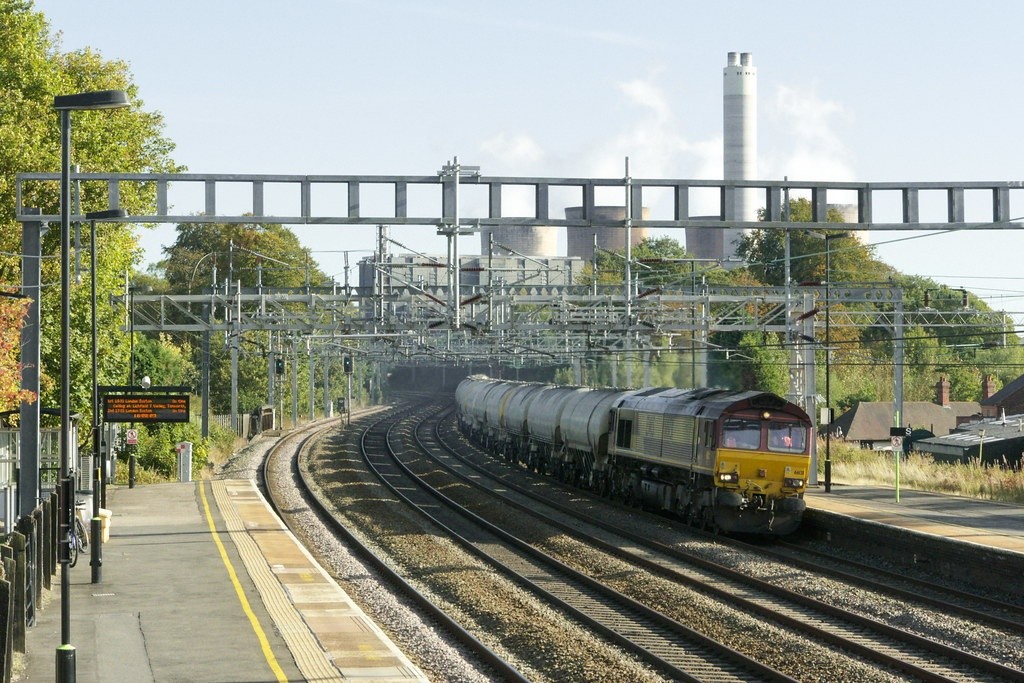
[57,499,92,568]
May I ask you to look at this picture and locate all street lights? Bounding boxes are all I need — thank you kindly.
[802,228,849,495]
[83,207,127,584]
[125,285,139,488]
[52,86,134,683]
[674,260,703,388]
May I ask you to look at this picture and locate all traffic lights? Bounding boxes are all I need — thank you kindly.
[275,358,285,374]
[343,355,354,374]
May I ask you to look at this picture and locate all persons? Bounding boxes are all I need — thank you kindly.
[771,429,795,446]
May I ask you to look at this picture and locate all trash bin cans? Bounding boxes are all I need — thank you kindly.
[338,397,345,413]
[98,508,112,543]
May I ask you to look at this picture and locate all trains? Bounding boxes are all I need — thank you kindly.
[455,370,813,539]
